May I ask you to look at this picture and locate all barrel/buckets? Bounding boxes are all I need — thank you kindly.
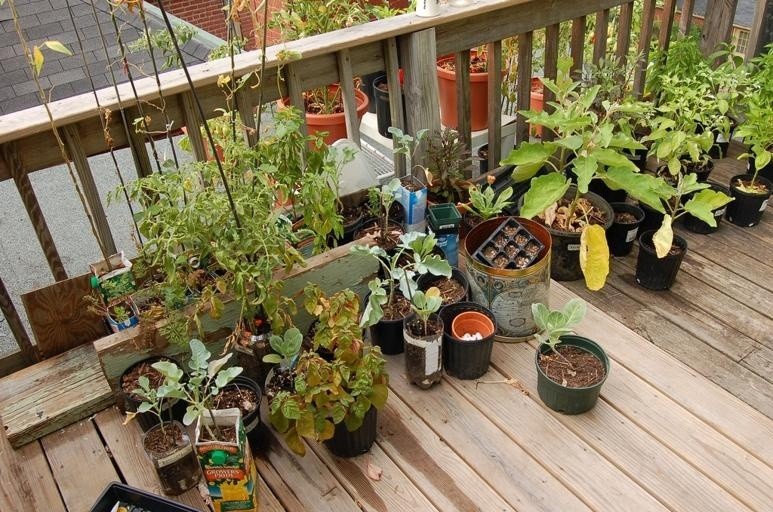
[464,217,552,344]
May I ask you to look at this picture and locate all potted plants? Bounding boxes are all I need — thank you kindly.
[354,235,451,355]
[369,6,422,137]
[397,232,447,289]
[386,123,431,234]
[403,290,443,387]
[424,129,478,236]
[436,41,507,131]
[531,299,611,415]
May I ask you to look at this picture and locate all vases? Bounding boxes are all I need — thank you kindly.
[522,75,564,140]
[438,304,497,381]
[360,69,389,111]
[416,266,470,316]
